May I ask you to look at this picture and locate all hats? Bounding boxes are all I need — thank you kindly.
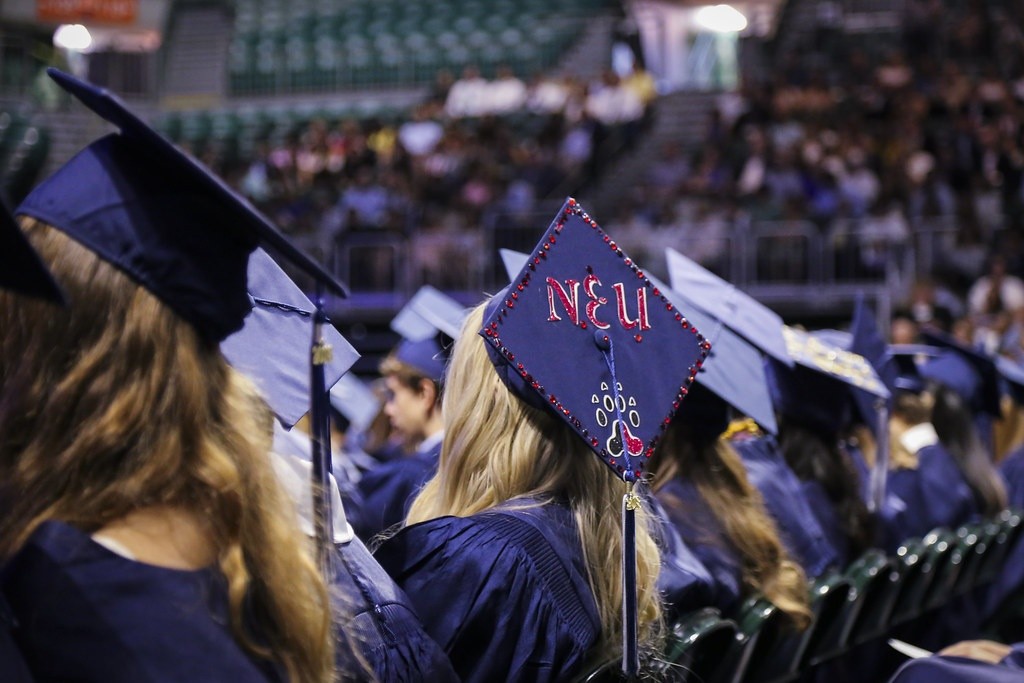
[660,247,794,435]
[475,196,712,674]
[15,64,349,578]
[643,269,779,435]
[218,247,361,429]
[785,323,890,402]
[1,208,64,305]
[890,320,1024,415]
[388,286,471,382]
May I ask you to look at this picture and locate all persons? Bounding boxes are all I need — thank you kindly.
[0,0,1024,683]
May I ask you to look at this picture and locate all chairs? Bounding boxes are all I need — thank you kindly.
[0,0,1024,683]
